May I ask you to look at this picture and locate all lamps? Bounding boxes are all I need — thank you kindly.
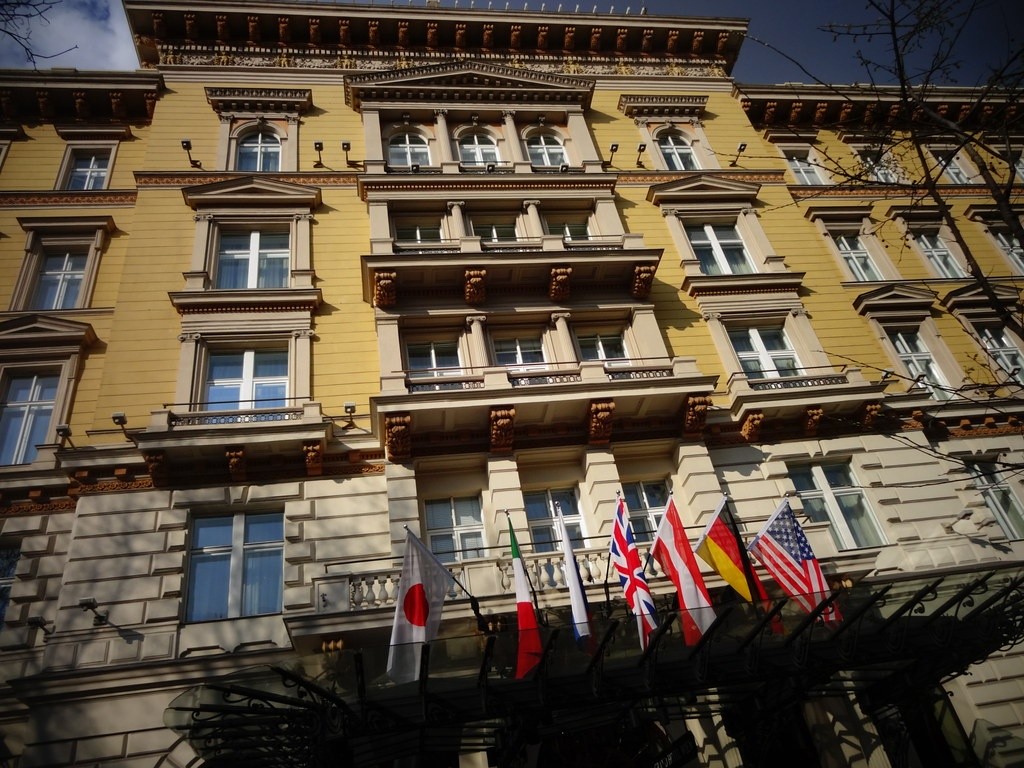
[602,144,619,168]
[78,597,109,626]
[344,402,356,430]
[636,144,647,166]
[941,508,974,535]
[559,163,569,173]
[906,371,926,392]
[486,162,496,174]
[342,142,354,165]
[55,424,76,449]
[730,143,747,167]
[977,516,997,531]
[112,412,130,440]
[313,142,324,168]
[410,162,420,174]
[986,364,1021,398]
[181,139,202,168]
[879,369,894,385]
[28,616,53,638]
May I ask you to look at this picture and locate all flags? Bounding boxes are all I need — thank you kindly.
[558,508,595,652]
[650,494,717,646]
[508,518,542,678]
[751,501,842,627]
[609,497,659,650]
[696,496,782,632]
[387,531,452,684]
